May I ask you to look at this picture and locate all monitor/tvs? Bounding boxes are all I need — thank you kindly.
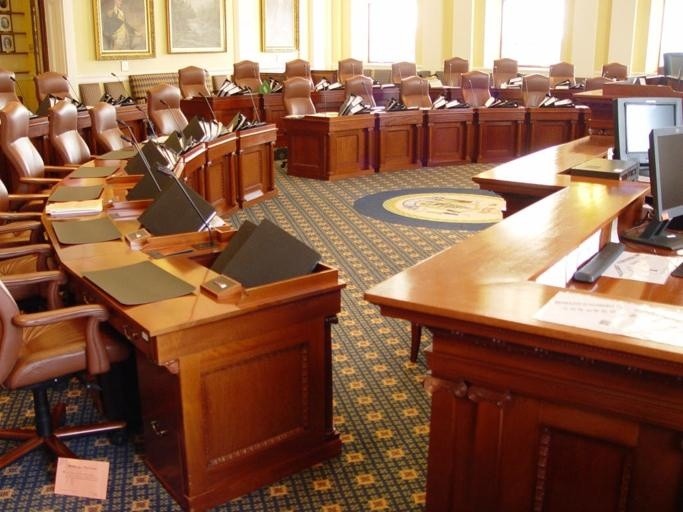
[612,98,682,178]
[620,128,683,251]
[663,52,683,82]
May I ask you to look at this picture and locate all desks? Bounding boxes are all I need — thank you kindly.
[32,119,349,512]
[179,85,682,186]
[360,130,682,512]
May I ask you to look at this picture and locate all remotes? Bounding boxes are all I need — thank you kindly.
[574,242,624,282]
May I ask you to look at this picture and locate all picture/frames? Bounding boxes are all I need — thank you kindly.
[164,0,228,55]
[92,0,156,61]
[259,0,299,52]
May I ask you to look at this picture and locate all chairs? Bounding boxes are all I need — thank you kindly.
[1,268,133,472]
[32,69,76,116]
[398,74,435,111]
[601,61,632,84]
[1,220,73,304]
[343,74,378,109]
[46,99,93,175]
[584,76,616,94]
[520,72,553,109]
[1,177,50,220]
[1,68,34,118]
[85,101,133,154]
[280,76,318,116]
[459,69,493,106]
[232,59,266,96]
[1,99,77,196]
[441,57,471,88]
[490,56,520,87]
[390,59,417,85]
[546,59,576,93]
[177,63,213,99]
[284,57,316,94]
[336,57,365,86]
[144,83,190,137]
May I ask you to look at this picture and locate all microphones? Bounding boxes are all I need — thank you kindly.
[10,71,216,247]
[231,62,630,123]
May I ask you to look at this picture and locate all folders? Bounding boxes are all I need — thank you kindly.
[269,78,283,93]
[433,96,470,109]
[183,116,223,147]
[100,92,135,107]
[126,162,217,236]
[216,78,242,97]
[210,220,321,289]
[125,130,193,175]
[385,97,407,111]
[508,76,523,87]
[315,77,343,92]
[554,80,575,91]
[338,92,372,116]
[36,94,87,115]
[485,96,519,108]
[225,112,246,132]
[538,94,574,108]
